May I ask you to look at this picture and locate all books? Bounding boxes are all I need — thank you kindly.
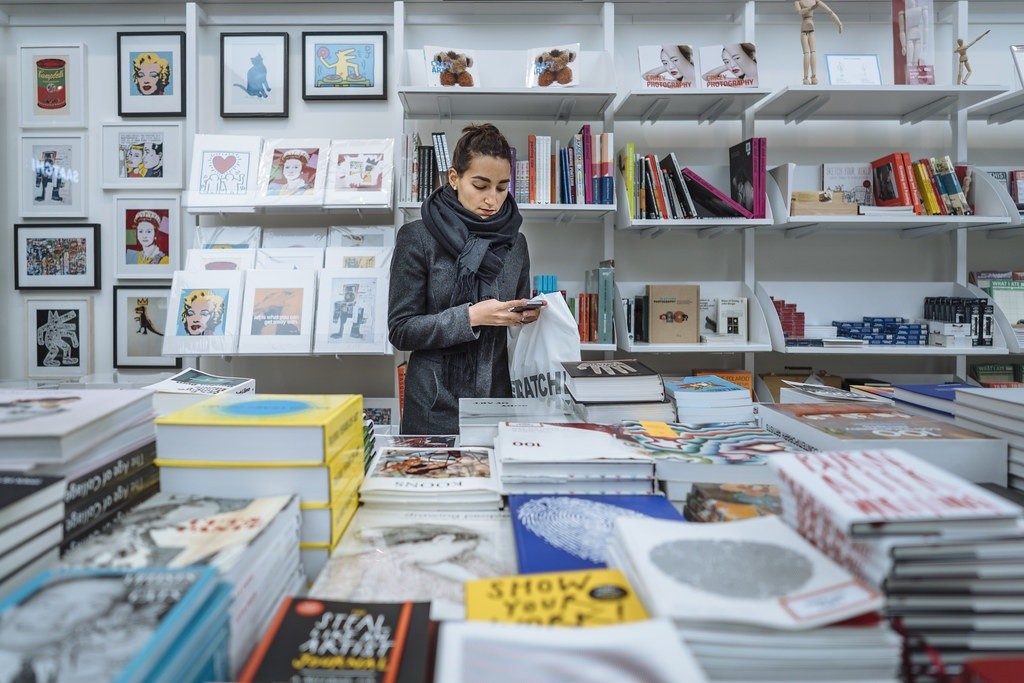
[969,271,1024,332]
[532,268,747,344]
[0,359,1024,683]
[402,124,614,205]
[769,152,973,216]
[987,171,1024,210]
[617,134,767,227]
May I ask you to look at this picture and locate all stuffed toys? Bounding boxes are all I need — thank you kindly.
[535,48,577,86]
[434,51,475,87]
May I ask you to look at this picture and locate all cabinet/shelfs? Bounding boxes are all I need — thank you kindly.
[395,0,1024,399]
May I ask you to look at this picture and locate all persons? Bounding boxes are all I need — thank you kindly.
[794,0,843,85]
[641,42,758,87]
[898,0,929,85]
[954,30,991,85]
[387,123,548,435]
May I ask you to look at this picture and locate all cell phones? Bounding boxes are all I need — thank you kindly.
[509,302,543,312]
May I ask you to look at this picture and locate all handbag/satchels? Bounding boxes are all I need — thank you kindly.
[507,291,582,398]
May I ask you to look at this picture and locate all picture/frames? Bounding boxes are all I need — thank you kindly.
[114,195,181,278]
[24,297,94,378]
[17,134,89,218]
[100,121,185,190]
[219,32,289,118]
[825,54,882,85]
[116,31,186,117]
[301,31,387,101]
[14,224,102,290]
[1009,45,1024,88]
[113,285,183,369]
[17,43,88,129]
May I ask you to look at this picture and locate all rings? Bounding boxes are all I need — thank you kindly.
[522,317,525,321]
